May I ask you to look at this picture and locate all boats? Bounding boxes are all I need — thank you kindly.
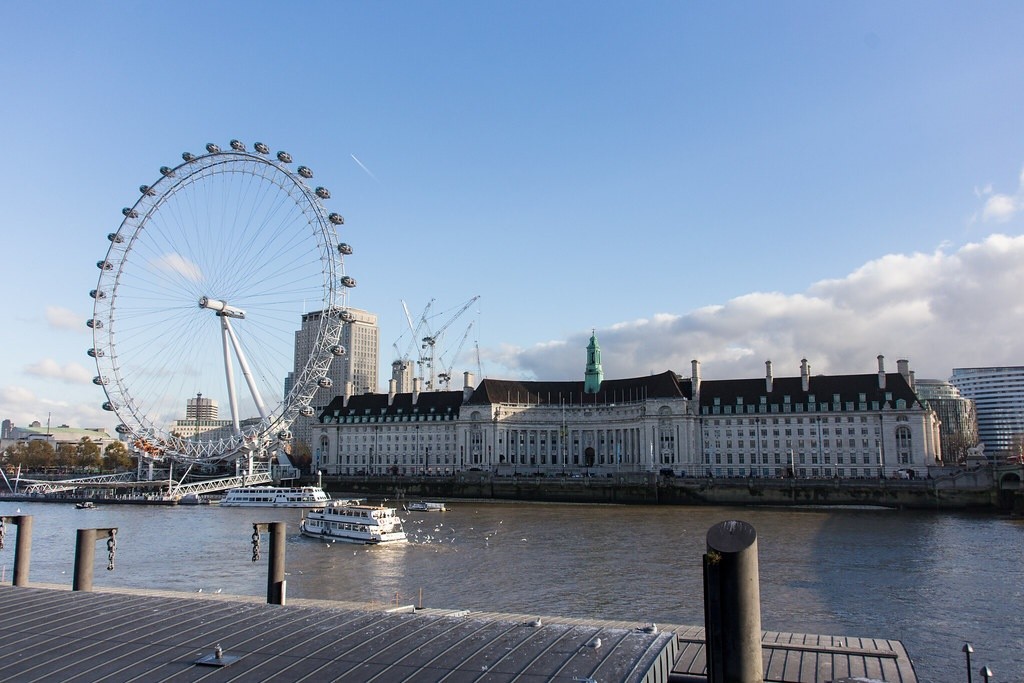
[408,501,452,512]
[219,485,360,508]
[298,504,409,545]
[177,492,211,505]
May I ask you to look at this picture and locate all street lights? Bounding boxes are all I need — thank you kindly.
[415,424,420,475]
[336,425,340,474]
[375,425,379,476]
